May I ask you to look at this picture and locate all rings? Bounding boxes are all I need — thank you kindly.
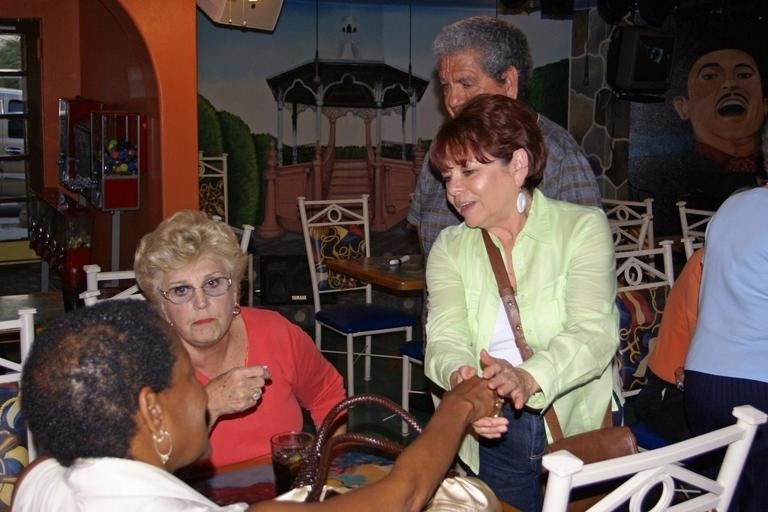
[252,391,261,401]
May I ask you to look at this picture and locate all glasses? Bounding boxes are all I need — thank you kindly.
[157,271,235,305]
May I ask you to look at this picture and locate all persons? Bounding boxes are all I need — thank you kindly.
[652,33,768,239]
[139,210,349,462]
[630,199,722,427]
[425,99,621,512]
[420,8,604,239]
[10,296,505,512]
[687,179,767,510]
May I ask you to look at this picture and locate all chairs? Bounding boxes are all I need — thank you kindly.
[296,193,415,437]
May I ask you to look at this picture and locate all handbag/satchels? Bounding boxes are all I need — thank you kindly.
[541,426,640,511]
[274,394,502,511]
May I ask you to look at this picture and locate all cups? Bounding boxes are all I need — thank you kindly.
[270,431,316,498]
